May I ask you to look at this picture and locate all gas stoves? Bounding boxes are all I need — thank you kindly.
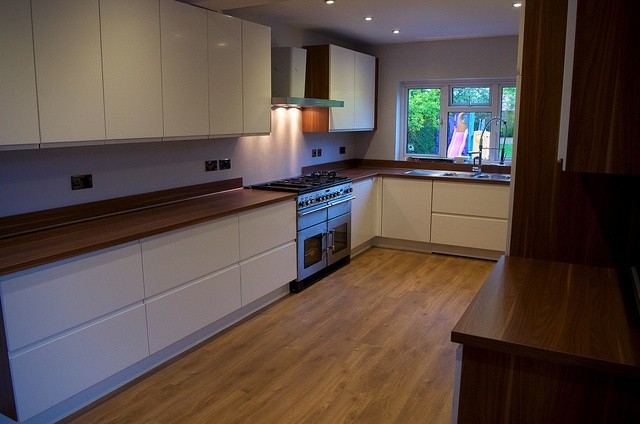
[244,171,353,210]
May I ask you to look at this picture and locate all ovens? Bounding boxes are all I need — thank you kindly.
[296,196,353,291]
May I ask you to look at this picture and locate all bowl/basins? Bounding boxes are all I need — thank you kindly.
[454,156,470,164]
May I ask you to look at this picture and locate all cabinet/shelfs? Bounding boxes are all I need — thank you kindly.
[348,177,377,259]
[31,0,108,150]
[0,240,152,424]
[100,0,164,146]
[209,9,245,141]
[242,19,272,136]
[381,176,432,254]
[430,180,511,261]
[1,0,43,152]
[162,0,209,141]
[301,43,378,133]
[237,197,298,320]
[139,213,245,370]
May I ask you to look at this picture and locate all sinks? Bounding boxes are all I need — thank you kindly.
[477,174,510,181]
[444,173,478,179]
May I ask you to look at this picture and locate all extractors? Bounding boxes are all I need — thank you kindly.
[271,44,345,108]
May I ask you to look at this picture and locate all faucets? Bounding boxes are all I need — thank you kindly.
[471,165,483,176]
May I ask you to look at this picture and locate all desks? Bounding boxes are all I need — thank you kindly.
[450,253,639,424]
